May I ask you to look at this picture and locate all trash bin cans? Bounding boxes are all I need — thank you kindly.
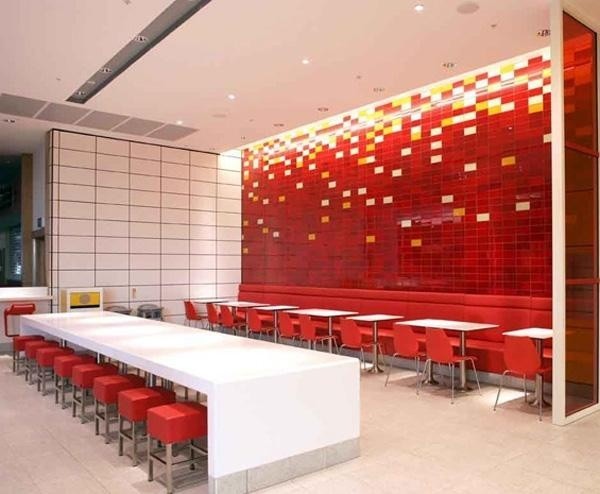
[105,306,132,316]
[137,304,164,320]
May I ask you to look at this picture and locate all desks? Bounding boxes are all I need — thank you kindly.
[19,311,360,494]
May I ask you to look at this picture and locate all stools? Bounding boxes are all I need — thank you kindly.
[12,335,207,494]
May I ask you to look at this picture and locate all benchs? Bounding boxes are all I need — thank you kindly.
[238,283,552,397]
